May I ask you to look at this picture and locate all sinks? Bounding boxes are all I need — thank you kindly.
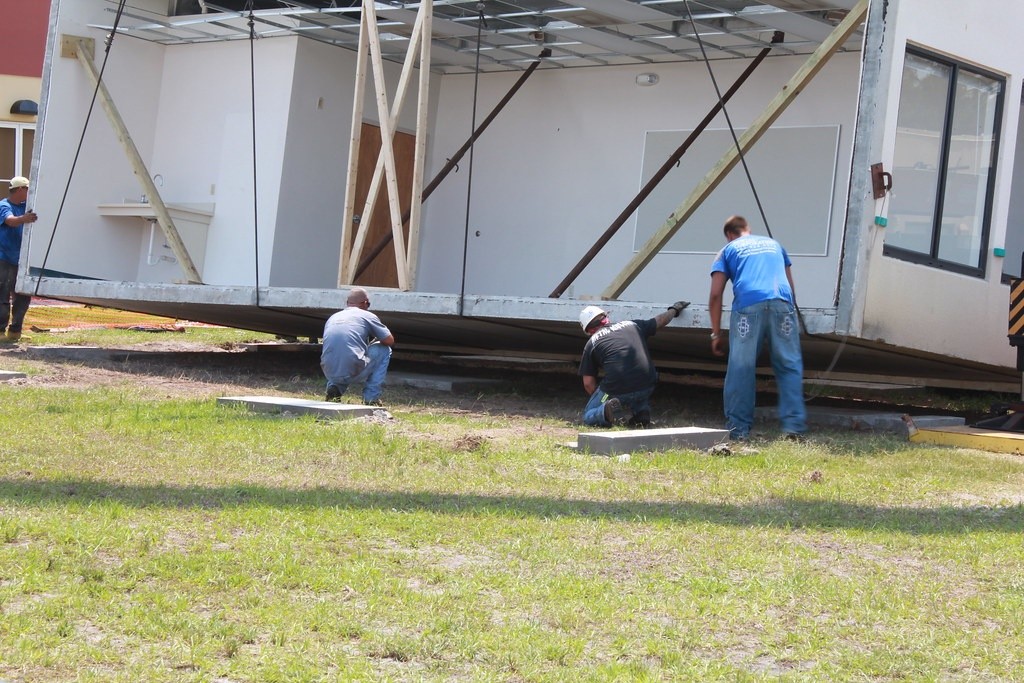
[98,203,196,221]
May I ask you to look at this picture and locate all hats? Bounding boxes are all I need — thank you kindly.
[9,176,29,189]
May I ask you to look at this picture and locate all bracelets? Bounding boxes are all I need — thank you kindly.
[711,331,723,340]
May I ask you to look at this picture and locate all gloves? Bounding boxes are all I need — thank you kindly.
[668,301,691,318]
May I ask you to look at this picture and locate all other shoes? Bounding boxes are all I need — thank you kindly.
[7,331,31,340]
[326,385,341,402]
[604,398,629,428]
[0,332,8,340]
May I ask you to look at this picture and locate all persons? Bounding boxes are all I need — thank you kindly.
[320,288,394,407]
[0,176,38,340]
[709,214,809,442]
[577,301,692,426]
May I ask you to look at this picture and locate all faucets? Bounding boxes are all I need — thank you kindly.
[146,174,164,202]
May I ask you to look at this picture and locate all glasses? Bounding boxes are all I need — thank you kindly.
[367,302,370,307]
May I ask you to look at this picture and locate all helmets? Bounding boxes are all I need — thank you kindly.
[579,306,607,335]
